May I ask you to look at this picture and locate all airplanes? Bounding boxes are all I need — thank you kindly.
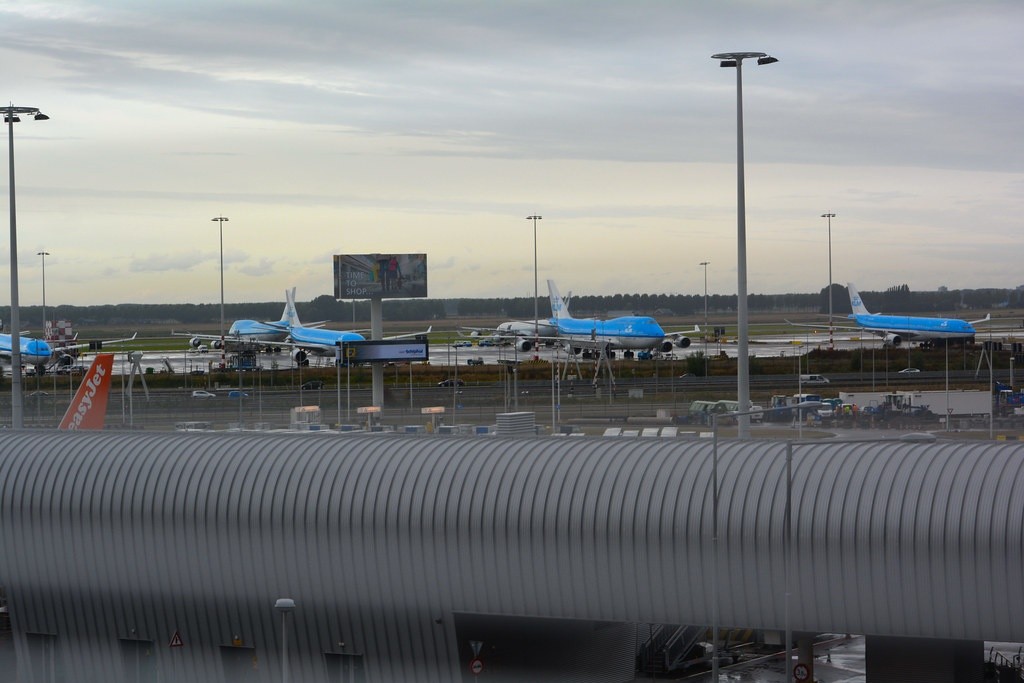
[170,286,332,353]
[463,279,700,361]
[462,289,574,352]
[0,328,137,369]
[190,288,433,366]
[783,283,993,349]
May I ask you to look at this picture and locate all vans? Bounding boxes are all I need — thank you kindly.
[800,374,831,386]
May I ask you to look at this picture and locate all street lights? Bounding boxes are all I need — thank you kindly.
[709,50,780,443]
[1,106,50,428]
[527,214,542,363]
[820,213,837,350]
[699,261,710,385]
[211,216,229,372]
[1008,357,1015,389]
[35,252,50,339]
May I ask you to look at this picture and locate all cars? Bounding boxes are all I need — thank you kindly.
[438,379,465,387]
[898,368,920,373]
[191,390,216,399]
[302,380,326,390]
[229,391,249,397]
